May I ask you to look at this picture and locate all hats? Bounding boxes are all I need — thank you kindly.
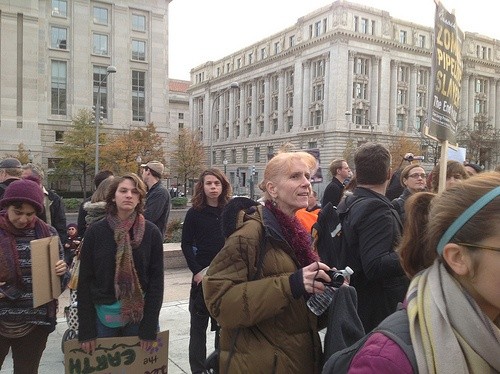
[67,223,78,230]
[141,160,164,174]
[0,180,44,215]
[0,157,22,168]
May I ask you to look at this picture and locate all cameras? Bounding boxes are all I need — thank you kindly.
[315,269,345,288]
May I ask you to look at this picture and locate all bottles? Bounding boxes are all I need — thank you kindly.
[306,266,354,316]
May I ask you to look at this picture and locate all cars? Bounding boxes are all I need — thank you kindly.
[170,188,184,197]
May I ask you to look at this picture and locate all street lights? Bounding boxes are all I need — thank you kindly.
[344,110,373,143]
[210,82,239,166]
[222,158,227,176]
[94,65,116,191]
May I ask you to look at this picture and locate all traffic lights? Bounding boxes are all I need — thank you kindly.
[99,106,103,125]
[91,106,96,124]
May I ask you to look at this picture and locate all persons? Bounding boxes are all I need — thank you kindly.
[318,158,500,215]
[321,170,500,374]
[203,149,351,374]
[338,144,409,333]
[181,167,237,374]
[0,179,73,374]
[77,174,163,374]
[0,158,171,263]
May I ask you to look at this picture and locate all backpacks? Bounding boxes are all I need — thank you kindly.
[311,196,371,270]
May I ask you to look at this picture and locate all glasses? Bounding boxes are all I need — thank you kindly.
[406,173,426,179]
[453,175,463,180]
[68,228,75,230]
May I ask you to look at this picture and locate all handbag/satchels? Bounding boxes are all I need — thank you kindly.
[29,235,62,308]
[201,351,219,374]
[93,292,145,328]
